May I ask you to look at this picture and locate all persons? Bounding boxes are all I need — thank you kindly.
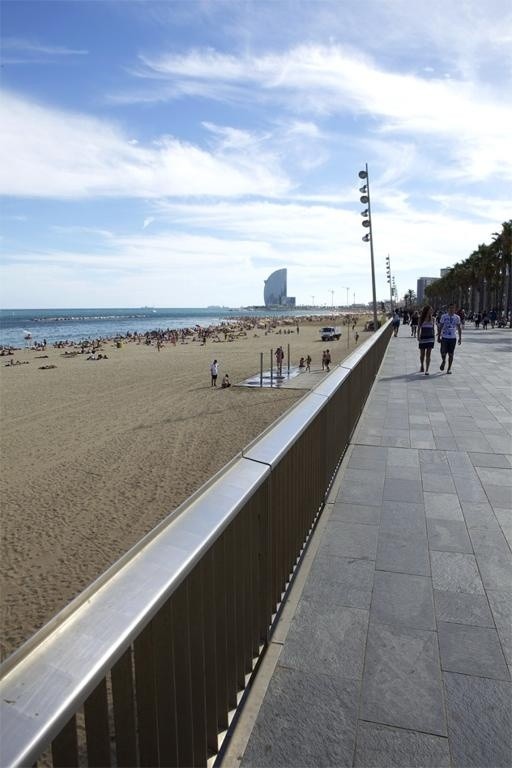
[210,359,220,387]
[299,355,311,371]
[223,312,360,344]
[392,307,512,339]
[113,321,248,351]
[222,374,231,387]
[1,334,113,370]
[416,303,441,376]
[437,303,463,374]
[321,349,332,371]
[275,346,284,371]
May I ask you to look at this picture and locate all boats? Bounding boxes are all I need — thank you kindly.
[24,334,32,338]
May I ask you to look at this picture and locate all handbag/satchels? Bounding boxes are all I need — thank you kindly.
[475,318,478,321]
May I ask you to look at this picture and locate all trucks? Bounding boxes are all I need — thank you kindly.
[319,327,342,341]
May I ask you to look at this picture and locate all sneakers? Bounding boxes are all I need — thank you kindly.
[421,366,429,375]
[440,362,451,374]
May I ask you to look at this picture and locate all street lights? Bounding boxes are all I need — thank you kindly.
[357,161,398,329]
